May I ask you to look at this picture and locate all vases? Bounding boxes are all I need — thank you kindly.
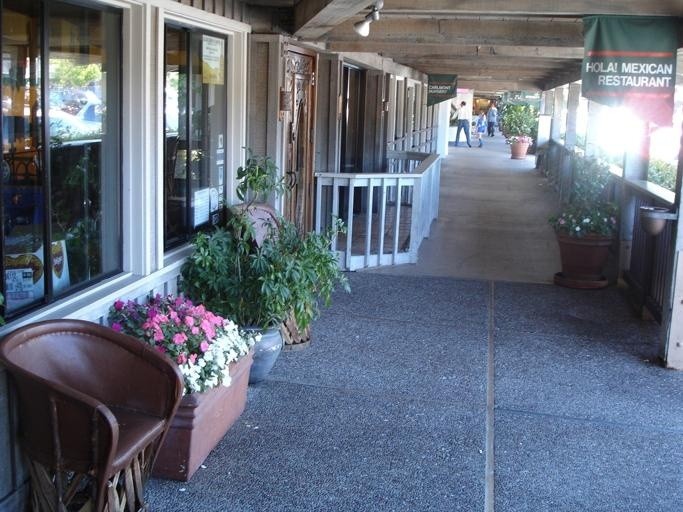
[638,203,670,237]
[554,227,620,291]
[150,347,257,484]
[509,143,532,160]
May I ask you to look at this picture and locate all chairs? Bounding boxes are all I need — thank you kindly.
[209,203,283,248]
[0,316,190,512]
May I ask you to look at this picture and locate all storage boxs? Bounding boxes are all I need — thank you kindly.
[4,226,69,314]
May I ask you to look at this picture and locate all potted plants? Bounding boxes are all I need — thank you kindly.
[179,135,352,388]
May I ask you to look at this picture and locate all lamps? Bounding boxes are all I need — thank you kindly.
[351,0,387,39]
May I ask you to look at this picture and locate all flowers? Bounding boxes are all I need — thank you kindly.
[545,152,618,238]
[104,290,265,396]
[506,131,534,146]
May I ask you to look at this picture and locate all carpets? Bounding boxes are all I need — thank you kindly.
[142,271,493,512]
[490,280,683,512]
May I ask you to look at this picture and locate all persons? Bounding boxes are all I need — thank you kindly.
[476,109,486,147]
[486,101,498,137]
[450,101,472,148]
